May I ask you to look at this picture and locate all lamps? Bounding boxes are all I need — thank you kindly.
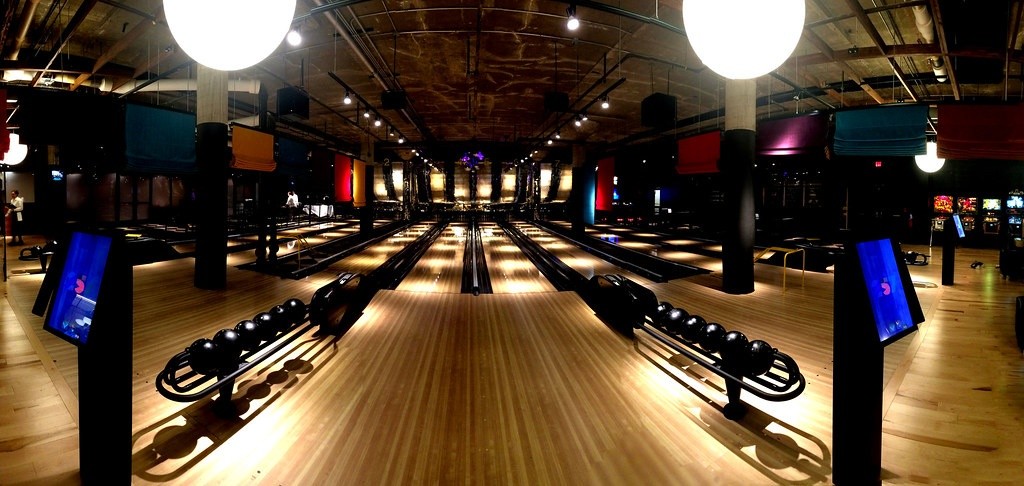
[343,90,439,172]
[509,96,611,171]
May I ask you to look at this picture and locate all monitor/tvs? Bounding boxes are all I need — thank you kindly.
[857,238,926,343]
[32,229,117,348]
[953,214,965,238]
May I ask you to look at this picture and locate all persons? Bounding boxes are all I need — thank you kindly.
[5,188,24,246]
[286,191,299,208]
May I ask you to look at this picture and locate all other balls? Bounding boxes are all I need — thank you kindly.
[652,301,773,374]
[187,297,306,374]
[905,250,916,262]
